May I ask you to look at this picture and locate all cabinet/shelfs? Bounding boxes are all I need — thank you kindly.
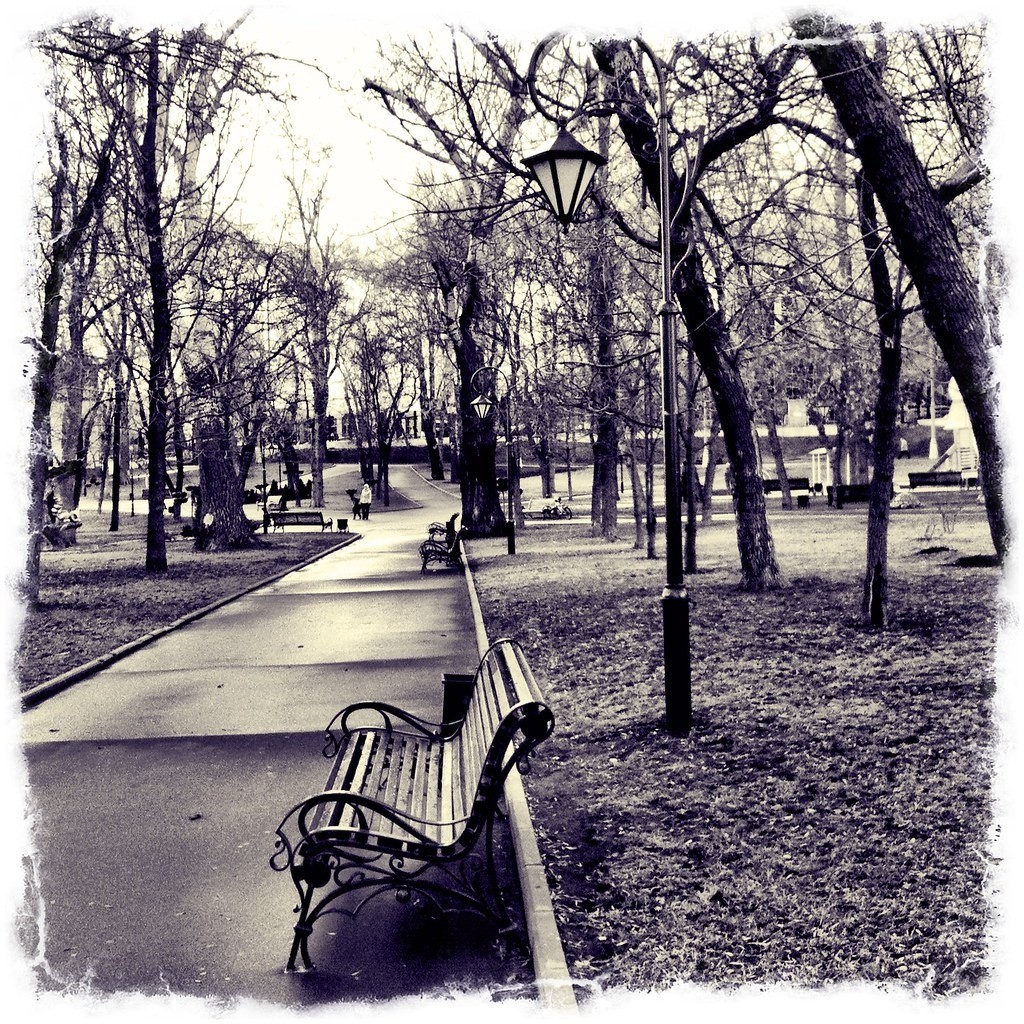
[761,478,815,495]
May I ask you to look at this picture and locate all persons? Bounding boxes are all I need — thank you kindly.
[852,468,867,485]
[45,485,56,522]
[682,460,703,502]
[513,488,528,519]
[898,438,911,459]
[351,484,371,520]
[555,497,568,512]
[90,475,97,494]
[724,467,732,494]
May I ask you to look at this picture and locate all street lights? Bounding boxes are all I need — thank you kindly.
[521,31,703,744]
[472,367,517,556]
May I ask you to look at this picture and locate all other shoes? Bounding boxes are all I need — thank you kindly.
[363,516,365,520]
[366,516,368,520]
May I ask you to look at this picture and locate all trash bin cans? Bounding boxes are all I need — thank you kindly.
[439,671,477,737]
[265,495,284,511]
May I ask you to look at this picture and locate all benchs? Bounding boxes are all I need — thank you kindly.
[270,639,555,974]
[908,471,965,492]
[521,497,557,521]
[270,511,332,534]
[418,513,470,575]
